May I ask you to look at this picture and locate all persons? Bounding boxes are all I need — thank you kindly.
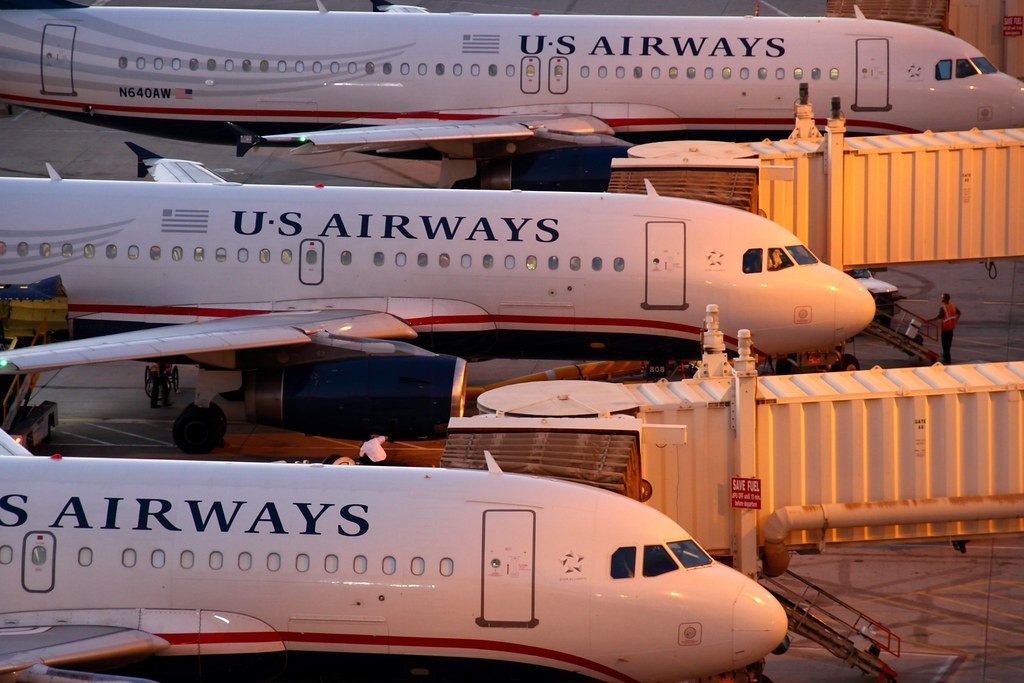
[926,292,963,364]
[147,361,178,407]
[359,434,390,465]
[643,348,676,382]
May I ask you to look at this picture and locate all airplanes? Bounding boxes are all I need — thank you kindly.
[0,143,880,457]
[0,414,790,683]
[0,0,1022,155]
